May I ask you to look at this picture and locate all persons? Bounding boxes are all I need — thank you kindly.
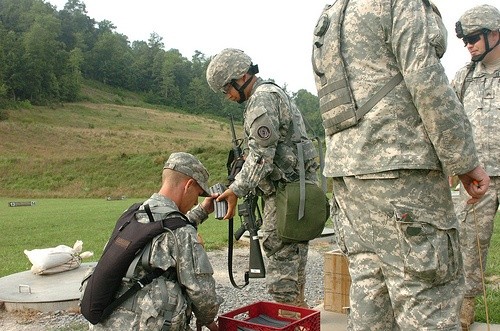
[93,152,224,331]
[311,0,491,331]
[216,47,321,309]
[450,4,500,331]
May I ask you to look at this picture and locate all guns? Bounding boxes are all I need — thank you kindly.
[226,116,266,279]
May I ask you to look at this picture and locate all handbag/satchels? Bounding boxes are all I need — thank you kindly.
[276,180,330,243]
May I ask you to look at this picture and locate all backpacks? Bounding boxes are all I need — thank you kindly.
[80,202,197,325]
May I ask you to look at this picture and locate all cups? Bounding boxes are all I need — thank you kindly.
[211,183,227,218]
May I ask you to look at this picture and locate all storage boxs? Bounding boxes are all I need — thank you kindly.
[218,301,321,331]
[323,249,352,314]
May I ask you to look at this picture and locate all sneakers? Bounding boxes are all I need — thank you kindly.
[459,297,474,331]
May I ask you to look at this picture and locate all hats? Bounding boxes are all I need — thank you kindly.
[163,152,212,197]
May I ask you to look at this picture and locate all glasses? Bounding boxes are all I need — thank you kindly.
[222,80,235,94]
[463,33,483,44]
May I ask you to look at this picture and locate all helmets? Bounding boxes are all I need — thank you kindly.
[455,4,500,39]
[206,48,251,93]
